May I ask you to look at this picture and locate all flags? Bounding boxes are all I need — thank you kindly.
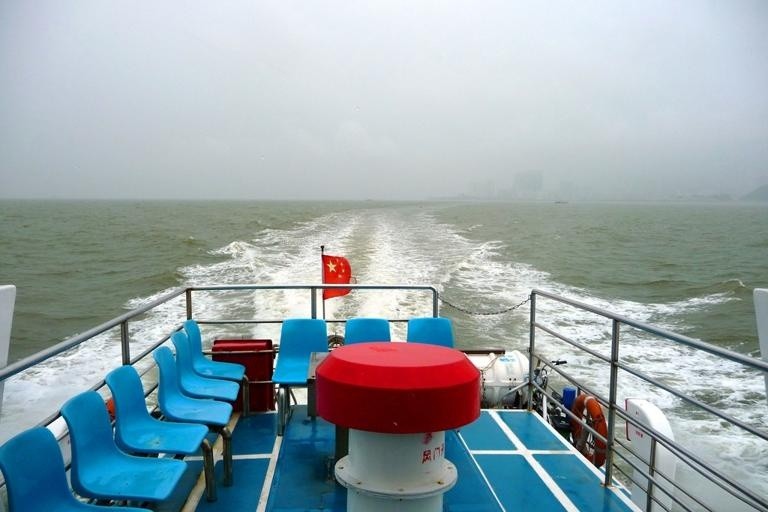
[321,254,353,300]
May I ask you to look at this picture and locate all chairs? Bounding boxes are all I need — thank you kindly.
[272,318,454,437]
[105,362,217,506]
[0,421,154,512]
[153,317,250,486]
[64,388,189,512]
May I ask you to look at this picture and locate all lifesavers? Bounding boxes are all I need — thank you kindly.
[571,395,607,467]
